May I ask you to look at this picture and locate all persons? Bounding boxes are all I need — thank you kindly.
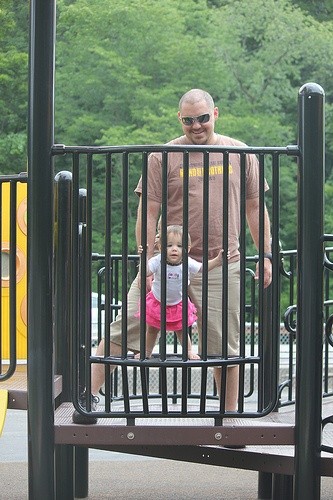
[134,226,231,359]
[71,89,273,448]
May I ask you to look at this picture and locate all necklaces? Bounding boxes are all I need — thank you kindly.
[166,260,182,266]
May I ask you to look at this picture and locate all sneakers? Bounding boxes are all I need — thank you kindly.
[72,392,102,424]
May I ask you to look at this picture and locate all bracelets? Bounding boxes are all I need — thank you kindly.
[263,253,272,265]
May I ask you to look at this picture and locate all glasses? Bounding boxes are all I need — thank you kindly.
[178,112,215,125]
